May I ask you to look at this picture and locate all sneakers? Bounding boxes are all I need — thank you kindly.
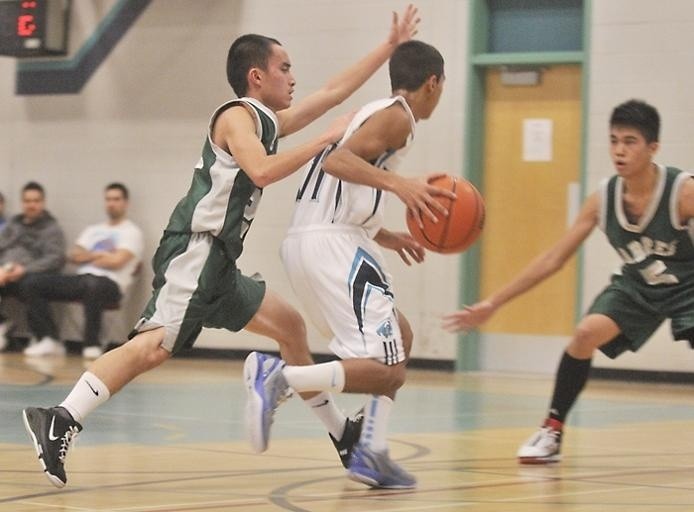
[347,441,418,489]
[242,351,294,453]
[22,406,83,488]
[517,426,562,463]
[82,345,102,360]
[328,406,365,470]
[23,336,67,357]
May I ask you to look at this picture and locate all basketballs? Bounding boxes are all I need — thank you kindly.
[406,174,486,253]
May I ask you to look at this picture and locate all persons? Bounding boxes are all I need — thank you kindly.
[440,101,694,463]
[22,1,419,490]
[22,183,144,359]
[0,184,61,354]
[244,41,459,489]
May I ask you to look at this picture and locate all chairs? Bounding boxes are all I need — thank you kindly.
[53,254,143,351]
